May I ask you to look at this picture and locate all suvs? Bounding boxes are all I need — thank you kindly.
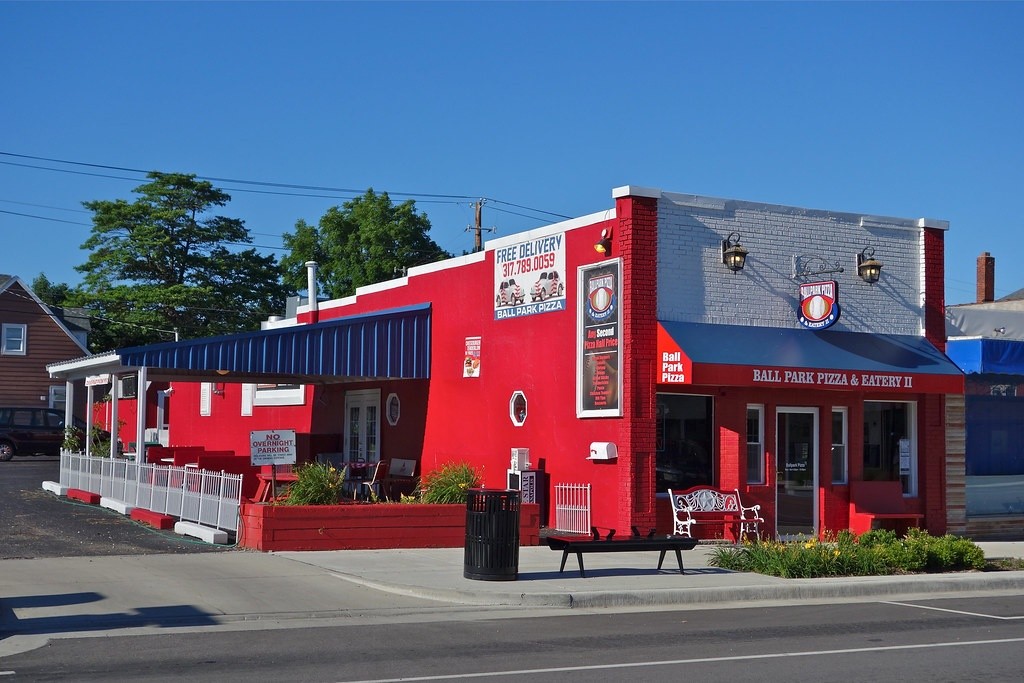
[0,404,123,463]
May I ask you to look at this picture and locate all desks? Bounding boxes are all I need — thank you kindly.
[123,452,148,457]
[184,463,198,468]
[257,473,300,502]
[340,462,389,500]
[161,458,174,464]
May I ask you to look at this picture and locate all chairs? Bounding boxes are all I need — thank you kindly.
[315,453,351,499]
[360,460,389,503]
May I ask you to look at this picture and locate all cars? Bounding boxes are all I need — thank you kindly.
[656,438,710,489]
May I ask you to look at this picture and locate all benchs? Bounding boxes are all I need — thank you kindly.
[190,456,261,498]
[668,485,764,545]
[546,526,699,579]
[170,450,235,488]
[848,481,925,543]
[147,446,205,485]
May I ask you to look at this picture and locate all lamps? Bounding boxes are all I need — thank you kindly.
[856,246,884,287]
[594,239,609,253]
[720,232,749,275]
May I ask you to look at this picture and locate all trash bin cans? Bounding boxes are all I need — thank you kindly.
[460,485,523,583]
